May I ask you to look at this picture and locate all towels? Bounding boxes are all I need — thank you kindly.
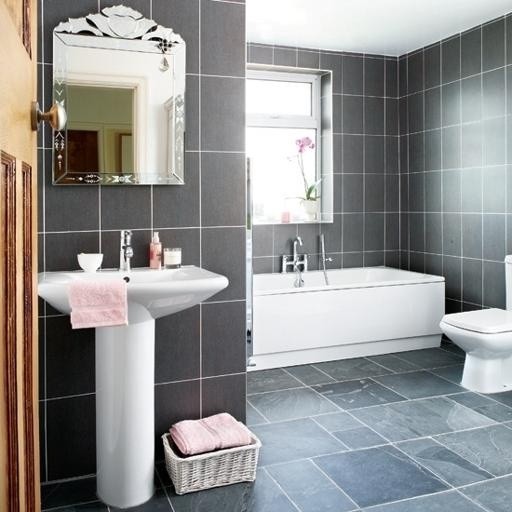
[68,279,128,329]
[168,413,252,456]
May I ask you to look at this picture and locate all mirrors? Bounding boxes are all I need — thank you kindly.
[52,33,186,187]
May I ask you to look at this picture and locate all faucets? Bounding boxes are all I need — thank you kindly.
[282,234,308,274]
[118,227,134,269]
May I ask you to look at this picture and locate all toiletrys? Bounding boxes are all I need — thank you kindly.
[148,231,162,269]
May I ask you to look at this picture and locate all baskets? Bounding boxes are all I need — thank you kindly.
[160,421,262,495]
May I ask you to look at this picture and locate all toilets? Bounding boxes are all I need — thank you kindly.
[440,256,511,396]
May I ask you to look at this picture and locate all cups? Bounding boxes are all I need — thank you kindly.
[163,247,182,270]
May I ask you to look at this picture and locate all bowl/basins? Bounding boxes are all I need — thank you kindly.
[76,252,104,272]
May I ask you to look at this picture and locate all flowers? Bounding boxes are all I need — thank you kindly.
[287,137,322,201]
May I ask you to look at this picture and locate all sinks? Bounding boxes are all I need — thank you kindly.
[36,265,229,318]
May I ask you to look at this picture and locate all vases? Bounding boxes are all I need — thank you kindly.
[302,200,317,220]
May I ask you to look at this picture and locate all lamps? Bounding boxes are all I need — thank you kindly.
[32,100,67,132]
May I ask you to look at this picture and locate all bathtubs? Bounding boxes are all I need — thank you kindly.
[252,265,443,372]
